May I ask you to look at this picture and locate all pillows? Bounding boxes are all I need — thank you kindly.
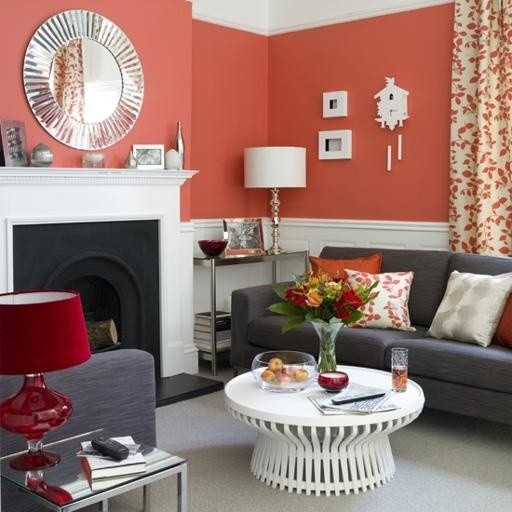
[308,252,512,348]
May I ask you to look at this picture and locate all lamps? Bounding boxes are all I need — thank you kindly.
[25,470,74,507]
[243,146,307,255]
[0,288,92,470]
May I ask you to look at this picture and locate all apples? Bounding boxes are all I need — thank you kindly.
[261,357,308,385]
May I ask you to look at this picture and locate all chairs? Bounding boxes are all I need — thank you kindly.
[0,349,157,512]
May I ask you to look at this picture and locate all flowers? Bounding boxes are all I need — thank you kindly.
[267,266,380,373]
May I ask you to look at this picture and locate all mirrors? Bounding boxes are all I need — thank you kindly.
[22,8,145,150]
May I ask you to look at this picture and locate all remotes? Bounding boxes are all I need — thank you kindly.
[92,434,129,459]
[331,390,385,405]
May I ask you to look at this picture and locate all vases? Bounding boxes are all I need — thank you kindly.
[310,321,345,374]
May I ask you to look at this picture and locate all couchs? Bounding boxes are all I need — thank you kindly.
[229,246,512,426]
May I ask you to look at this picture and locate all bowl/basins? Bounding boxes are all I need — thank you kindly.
[198,239,228,257]
[318,370,350,393]
[251,351,319,394]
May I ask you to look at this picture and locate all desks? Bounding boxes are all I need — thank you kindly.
[193,249,310,376]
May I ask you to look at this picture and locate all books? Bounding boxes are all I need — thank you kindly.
[87,475,148,491]
[309,380,400,416]
[194,311,232,354]
[76,435,147,480]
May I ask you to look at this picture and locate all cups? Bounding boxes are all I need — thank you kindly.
[391,348,409,393]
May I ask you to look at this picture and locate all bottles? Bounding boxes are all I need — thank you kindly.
[124,150,138,169]
[165,148,179,169]
[174,120,185,170]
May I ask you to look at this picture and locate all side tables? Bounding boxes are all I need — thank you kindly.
[0,428,188,512]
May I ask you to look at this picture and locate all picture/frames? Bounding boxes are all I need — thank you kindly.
[223,218,267,256]
[0,119,30,167]
[132,144,165,170]
